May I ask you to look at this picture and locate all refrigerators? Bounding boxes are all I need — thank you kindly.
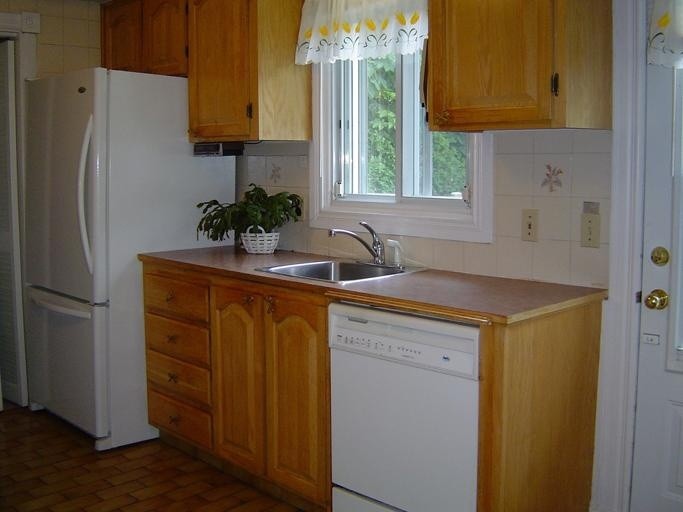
[24,67,239,452]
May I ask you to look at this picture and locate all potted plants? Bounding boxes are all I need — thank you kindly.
[195,182,303,254]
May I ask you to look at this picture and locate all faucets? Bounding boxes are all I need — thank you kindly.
[327,221,385,264]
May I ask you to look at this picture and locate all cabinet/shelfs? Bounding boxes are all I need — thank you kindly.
[98,1,188,78]
[186,0,313,144]
[427,1,612,133]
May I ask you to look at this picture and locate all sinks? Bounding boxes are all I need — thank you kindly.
[254,261,430,284]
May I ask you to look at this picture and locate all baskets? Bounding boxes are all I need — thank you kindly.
[240,225,279,255]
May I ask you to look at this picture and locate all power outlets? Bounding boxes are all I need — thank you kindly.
[520,209,539,242]
[580,214,600,248]
